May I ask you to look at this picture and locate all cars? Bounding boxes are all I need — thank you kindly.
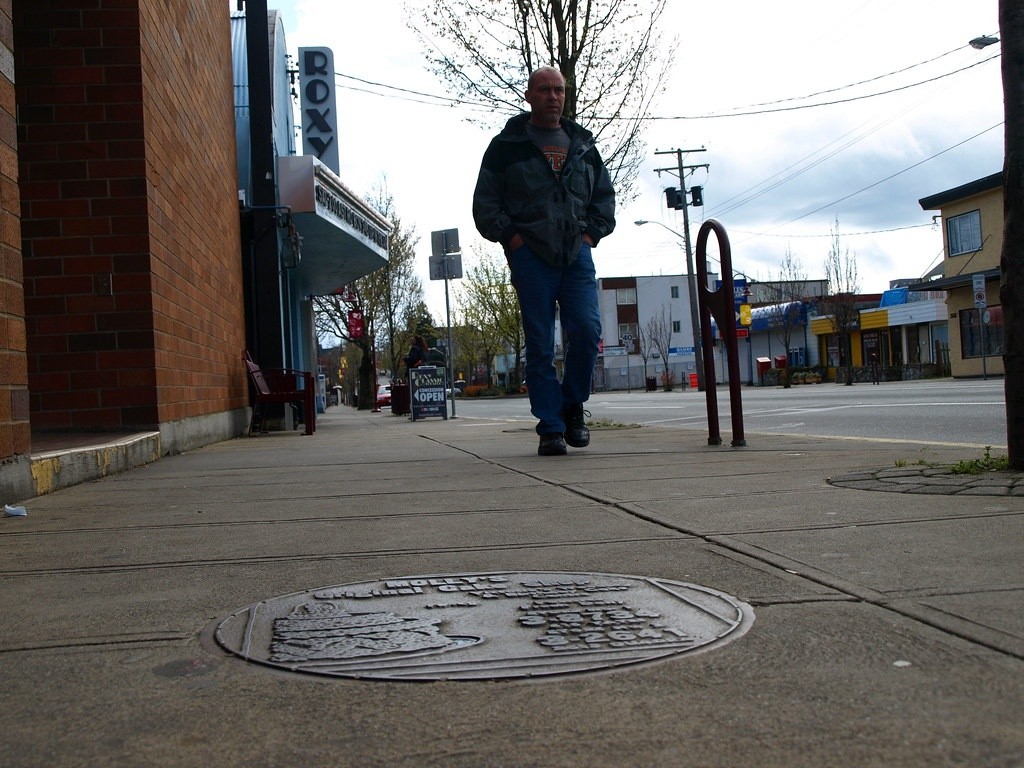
[376,384,393,411]
[446,387,462,398]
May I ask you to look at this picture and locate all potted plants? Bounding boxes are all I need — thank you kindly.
[792,372,822,383]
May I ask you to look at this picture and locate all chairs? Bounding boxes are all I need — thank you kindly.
[239,348,316,437]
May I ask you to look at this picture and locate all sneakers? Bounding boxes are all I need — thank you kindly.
[560,403,591,448]
[537,432,567,456]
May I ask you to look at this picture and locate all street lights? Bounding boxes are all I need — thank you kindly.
[633,219,706,392]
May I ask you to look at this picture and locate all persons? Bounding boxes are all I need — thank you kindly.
[472,66,616,457]
[402,334,428,420]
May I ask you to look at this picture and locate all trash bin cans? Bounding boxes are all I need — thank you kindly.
[388,381,411,416]
[646,376,657,392]
